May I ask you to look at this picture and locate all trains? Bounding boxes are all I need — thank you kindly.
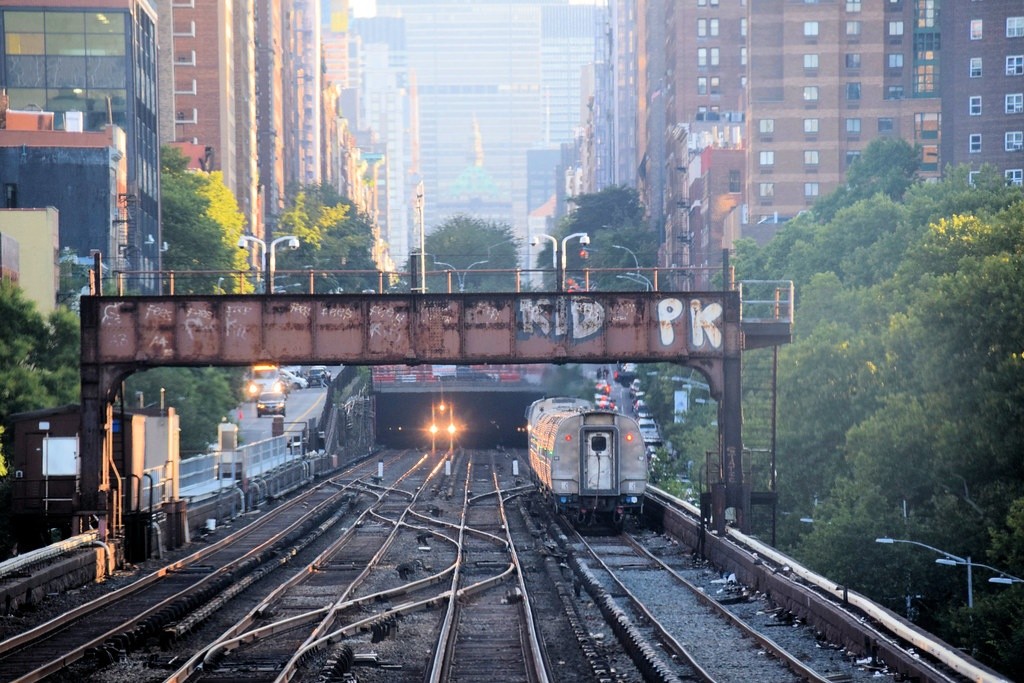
[525,395,650,530]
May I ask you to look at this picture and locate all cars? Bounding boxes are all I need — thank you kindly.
[594,379,618,411]
[629,380,663,461]
[256,390,287,417]
[246,365,332,402]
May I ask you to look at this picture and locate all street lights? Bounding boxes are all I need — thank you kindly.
[530,233,558,291]
[876,538,974,611]
[562,232,591,292]
[237,235,266,292]
[269,235,300,293]
[612,245,640,289]
